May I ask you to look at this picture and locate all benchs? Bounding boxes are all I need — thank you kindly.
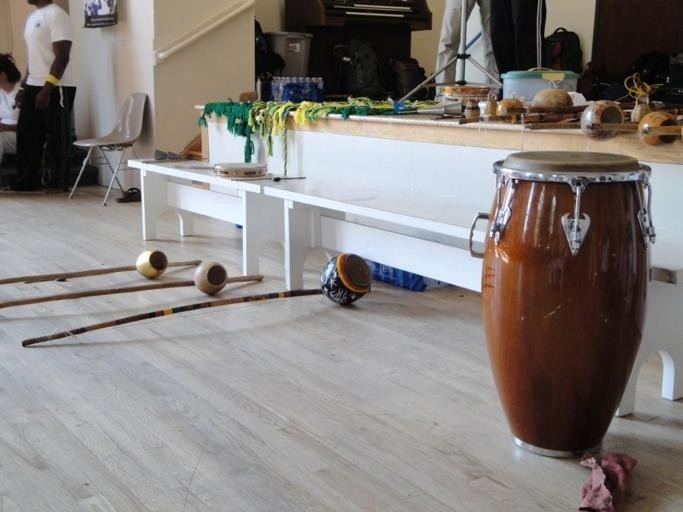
[127,94,682,417]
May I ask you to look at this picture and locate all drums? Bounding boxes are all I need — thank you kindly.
[469,151,656,459]
[320,253,370,305]
[214,162,266,178]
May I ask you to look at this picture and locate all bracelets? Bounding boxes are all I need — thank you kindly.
[45,73,59,87]
[17,86,25,91]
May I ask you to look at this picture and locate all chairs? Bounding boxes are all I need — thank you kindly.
[69,94,147,206]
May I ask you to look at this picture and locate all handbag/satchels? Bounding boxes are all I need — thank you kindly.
[541,28,582,74]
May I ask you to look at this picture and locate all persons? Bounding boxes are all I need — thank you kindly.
[10,0,79,191]
[0,53,49,166]
[490,0,547,101]
[434,0,500,111]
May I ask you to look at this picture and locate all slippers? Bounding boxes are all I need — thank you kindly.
[117,187,140,203]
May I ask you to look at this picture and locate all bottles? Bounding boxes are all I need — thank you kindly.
[271,77,324,101]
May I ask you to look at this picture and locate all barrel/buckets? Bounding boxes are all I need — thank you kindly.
[261,32,314,76]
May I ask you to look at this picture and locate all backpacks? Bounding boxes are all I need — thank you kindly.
[387,49,435,101]
[326,45,394,100]
